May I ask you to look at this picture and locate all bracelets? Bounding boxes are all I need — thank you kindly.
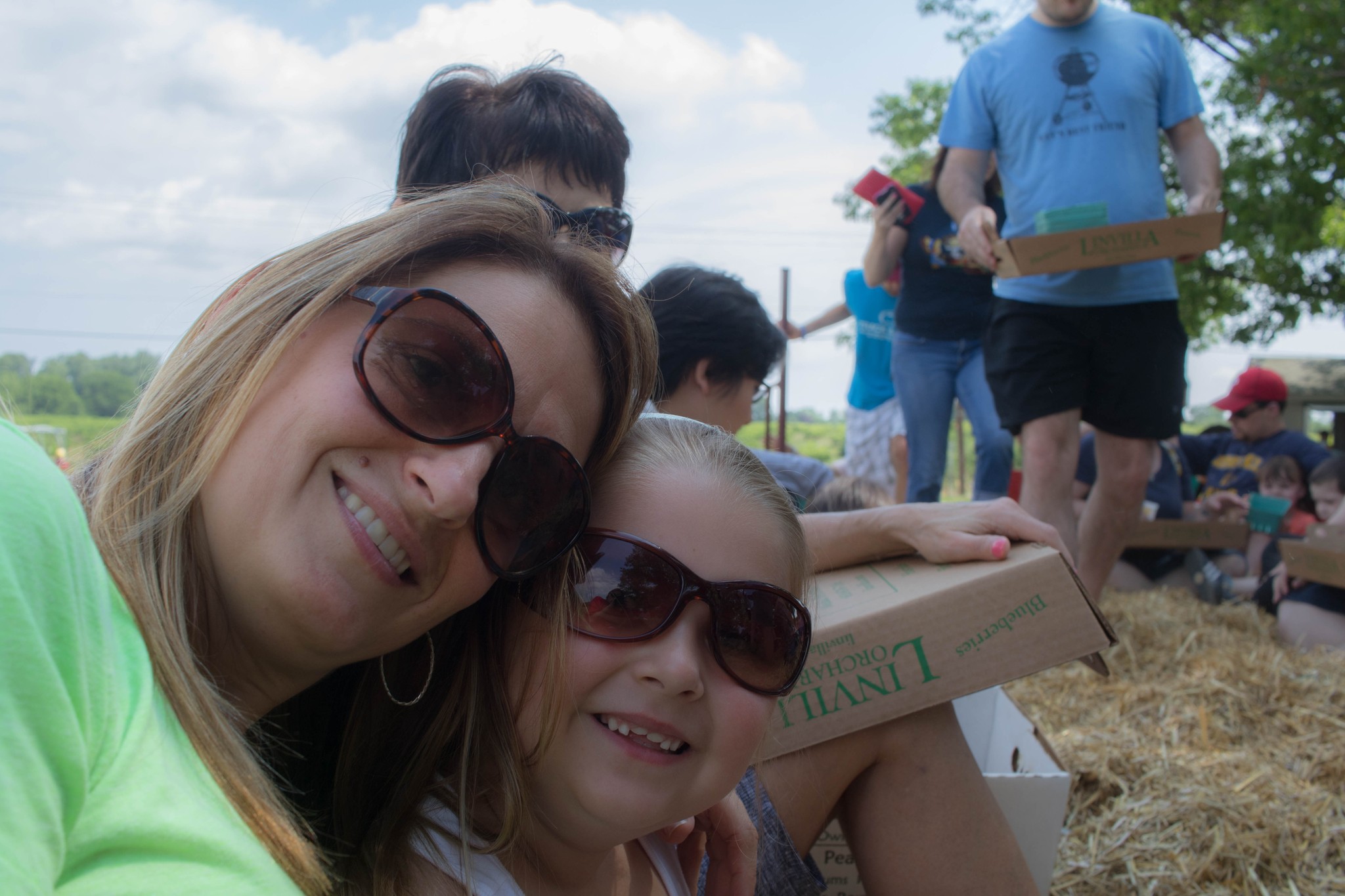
[801,326,806,334]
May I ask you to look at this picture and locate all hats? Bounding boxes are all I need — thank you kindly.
[1212,367,1288,411]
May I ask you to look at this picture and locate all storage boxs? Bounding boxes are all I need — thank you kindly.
[1126,520,1250,548]
[812,685,1076,896]
[990,210,1231,279]
[752,545,1118,767]
[1278,538,1345,587]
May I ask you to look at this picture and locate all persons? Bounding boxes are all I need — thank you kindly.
[937,0,1225,607]
[0,64,1345,896]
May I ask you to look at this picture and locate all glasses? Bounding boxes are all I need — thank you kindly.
[1234,402,1269,417]
[519,525,812,697]
[746,372,770,403]
[500,185,632,268]
[344,283,591,580]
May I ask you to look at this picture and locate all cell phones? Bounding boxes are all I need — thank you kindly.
[872,185,911,224]
